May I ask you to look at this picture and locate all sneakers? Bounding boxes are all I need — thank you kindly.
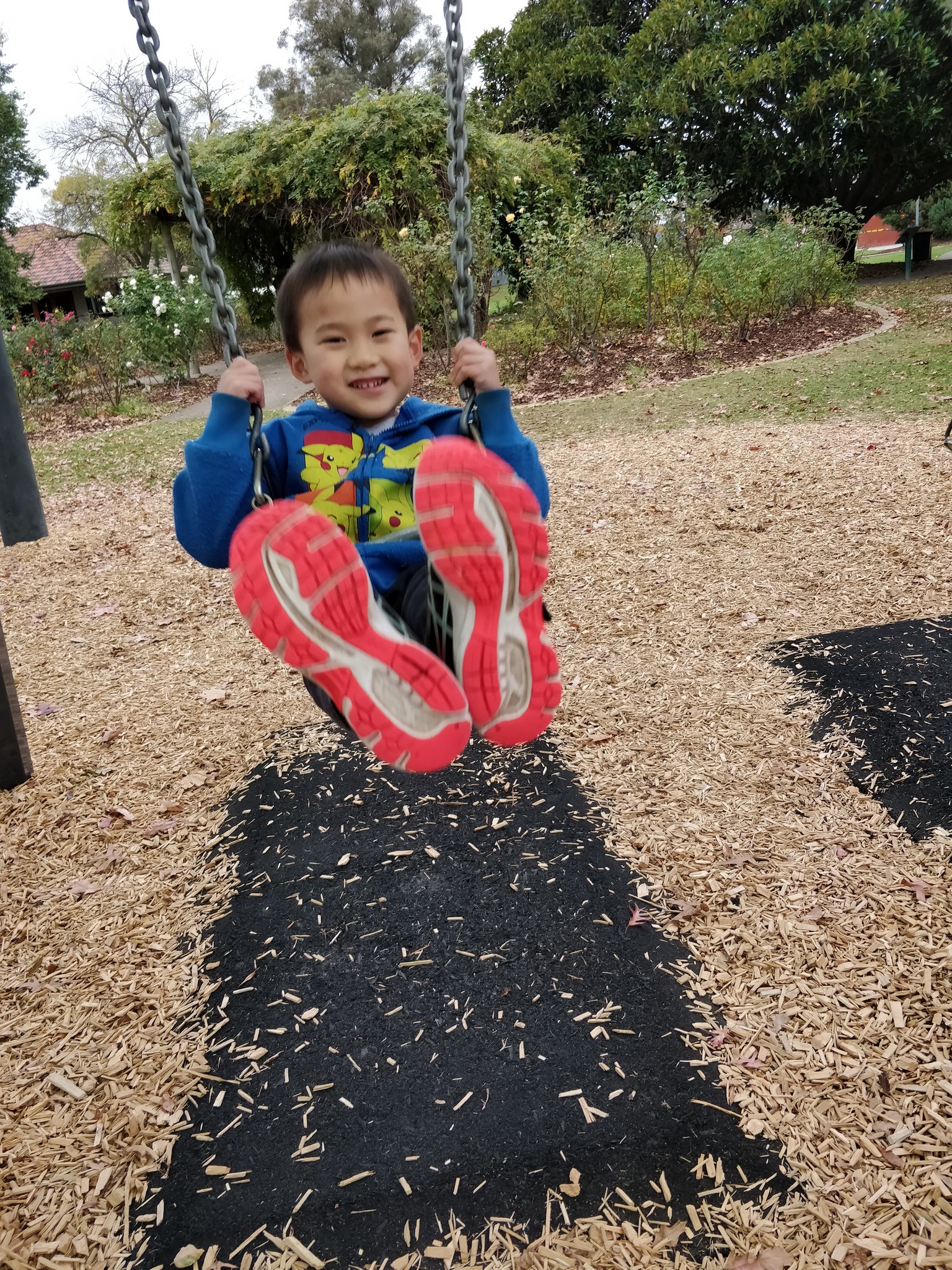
[411,435,564,746]
[229,500,470,776]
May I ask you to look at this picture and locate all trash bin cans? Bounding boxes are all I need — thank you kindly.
[913,229,934,261]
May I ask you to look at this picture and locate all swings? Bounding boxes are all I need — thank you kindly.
[127,0,555,735]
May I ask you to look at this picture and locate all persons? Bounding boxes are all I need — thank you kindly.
[173,242,562,773]
